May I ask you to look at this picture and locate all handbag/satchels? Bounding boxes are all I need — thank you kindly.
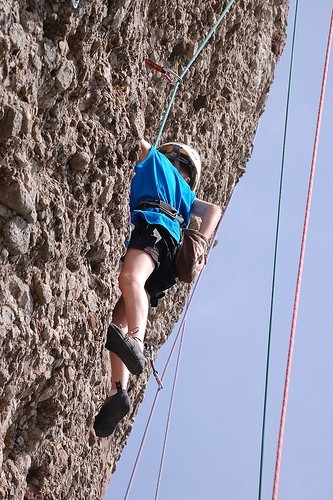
[175,226,209,283]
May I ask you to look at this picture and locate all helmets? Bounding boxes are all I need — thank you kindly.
[156,141,201,192]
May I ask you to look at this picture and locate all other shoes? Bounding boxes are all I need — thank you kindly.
[105,323,148,375]
[93,380,129,437]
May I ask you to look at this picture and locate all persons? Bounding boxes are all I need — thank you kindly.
[93,139,202,438]
